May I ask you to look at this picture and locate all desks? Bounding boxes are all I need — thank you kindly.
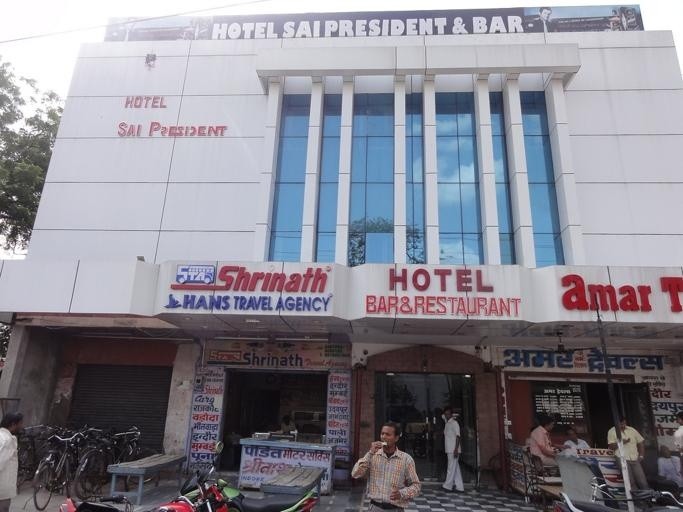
[537,485,562,512]
[261,467,327,505]
[106,454,187,505]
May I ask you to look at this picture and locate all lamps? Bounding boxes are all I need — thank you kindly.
[422,359,428,372]
[557,333,564,353]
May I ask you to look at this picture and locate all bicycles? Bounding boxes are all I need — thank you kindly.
[587,474,683,509]
[15,423,161,511]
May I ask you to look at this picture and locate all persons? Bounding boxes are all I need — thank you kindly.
[607,415,651,489]
[562,427,590,450]
[441,406,464,493]
[533,8,558,33]
[279,416,296,433]
[528,415,560,475]
[672,409,683,455]
[0,411,24,512]
[658,445,683,491]
[351,422,422,512]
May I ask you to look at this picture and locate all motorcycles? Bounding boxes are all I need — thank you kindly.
[173,440,323,512]
[57,468,231,512]
[551,490,683,512]
